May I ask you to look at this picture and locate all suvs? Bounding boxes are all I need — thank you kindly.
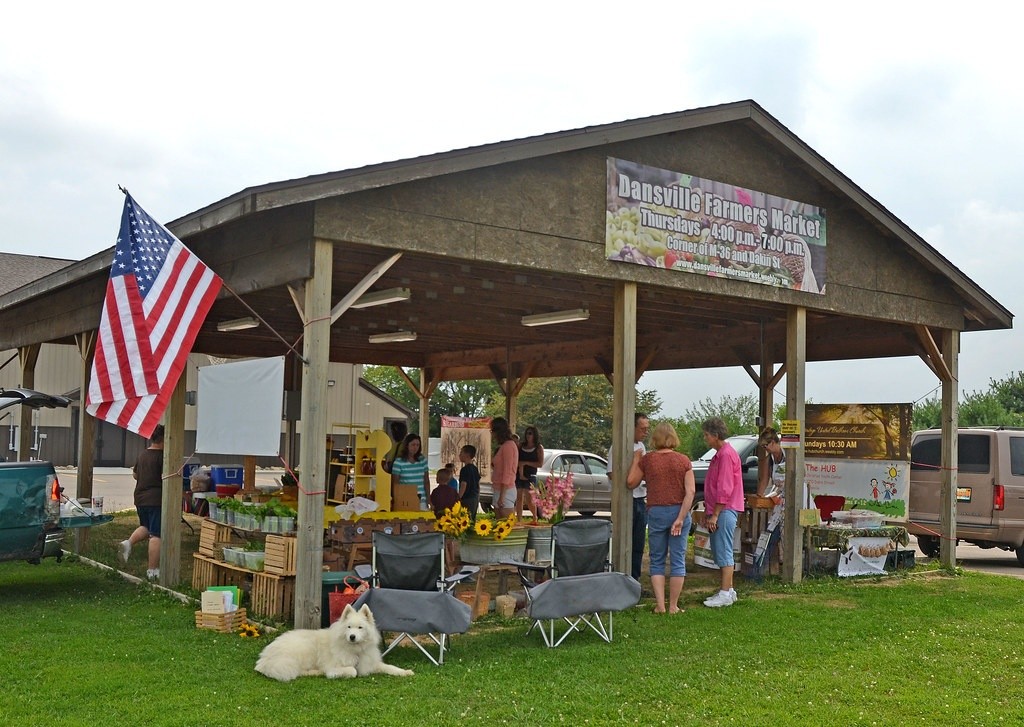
[906,424,1024,564]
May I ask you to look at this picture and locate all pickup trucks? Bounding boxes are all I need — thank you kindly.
[0,458,65,565]
[689,432,787,518]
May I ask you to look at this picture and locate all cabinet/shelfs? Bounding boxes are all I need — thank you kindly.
[192,518,298,580]
[352,430,398,512]
[325,423,370,506]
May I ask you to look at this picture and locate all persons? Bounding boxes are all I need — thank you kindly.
[607,412,649,579]
[431,469,460,520]
[488,416,519,521]
[627,422,695,613]
[459,445,480,519]
[759,427,817,510]
[385,421,408,466]
[391,434,431,510]
[117,424,165,579]
[511,426,544,522]
[446,464,459,491]
[701,417,745,608]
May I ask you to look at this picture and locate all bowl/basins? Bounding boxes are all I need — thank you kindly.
[455,529,528,564]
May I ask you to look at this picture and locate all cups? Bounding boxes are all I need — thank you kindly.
[91,496,104,516]
[527,548,535,564]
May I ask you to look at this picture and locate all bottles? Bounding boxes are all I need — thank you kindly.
[357,490,375,502]
[363,457,386,475]
[339,446,355,464]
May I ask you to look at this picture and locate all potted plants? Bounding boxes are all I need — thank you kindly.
[281,472,299,501]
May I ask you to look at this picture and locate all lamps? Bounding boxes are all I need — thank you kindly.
[350,286,412,308]
[369,331,417,345]
[520,308,591,327]
[217,317,260,332]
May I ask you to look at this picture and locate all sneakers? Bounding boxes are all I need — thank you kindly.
[703,593,733,607]
[706,591,738,601]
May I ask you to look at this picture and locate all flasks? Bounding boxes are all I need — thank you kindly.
[802,482,810,510]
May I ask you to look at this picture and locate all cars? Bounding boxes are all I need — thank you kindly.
[476,448,612,518]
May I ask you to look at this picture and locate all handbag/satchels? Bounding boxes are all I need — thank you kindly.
[329,576,370,625]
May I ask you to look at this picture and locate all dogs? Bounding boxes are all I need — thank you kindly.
[254,602,415,681]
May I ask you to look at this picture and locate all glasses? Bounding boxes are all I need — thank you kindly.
[761,442,771,449]
[526,431,533,435]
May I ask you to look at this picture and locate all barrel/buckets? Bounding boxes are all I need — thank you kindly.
[329,592,361,624]
[455,590,491,619]
[523,526,551,562]
[496,595,516,619]
[480,494,493,513]
[888,550,916,567]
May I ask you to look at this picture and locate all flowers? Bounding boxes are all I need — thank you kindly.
[432,501,515,544]
[528,461,581,524]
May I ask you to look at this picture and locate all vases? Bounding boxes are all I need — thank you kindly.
[458,527,529,564]
[524,526,555,561]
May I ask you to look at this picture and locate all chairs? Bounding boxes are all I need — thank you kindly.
[814,495,846,525]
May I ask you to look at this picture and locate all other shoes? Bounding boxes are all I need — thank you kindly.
[118,540,130,563]
[147,569,159,579]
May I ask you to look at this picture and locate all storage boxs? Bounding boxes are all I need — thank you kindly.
[350,529,482,665]
[183,456,202,491]
[339,454,355,464]
[198,520,233,559]
[226,510,233,526]
[195,608,246,634]
[242,514,251,530]
[222,547,245,566]
[234,494,247,503]
[499,516,642,649]
[392,517,428,533]
[740,507,773,545]
[887,549,916,569]
[332,449,344,458]
[250,493,284,503]
[216,508,226,523]
[816,550,837,568]
[233,512,243,528]
[260,516,279,533]
[209,502,217,521]
[252,574,295,621]
[741,542,779,578]
[393,483,421,512]
[358,518,401,536]
[250,518,260,529]
[328,593,363,625]
[192,558,245,594]
[427,519,437,533]
[327,518,373,543]
[215,483,240,498]
[747,496,775,509]
[209,463,244,492]
[245,552,265,572]
[797,509,821,526]
[264,534,297,577]
[278,517,294,532]
[458,590,490,620]
[832,510,885,528]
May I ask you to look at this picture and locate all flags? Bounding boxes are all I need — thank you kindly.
[85,194,224,439]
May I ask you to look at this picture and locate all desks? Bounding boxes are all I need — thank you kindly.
[341,543,373,573]
[803,526,911,571]
[473,565,520,620]
[692,509,747,572]
